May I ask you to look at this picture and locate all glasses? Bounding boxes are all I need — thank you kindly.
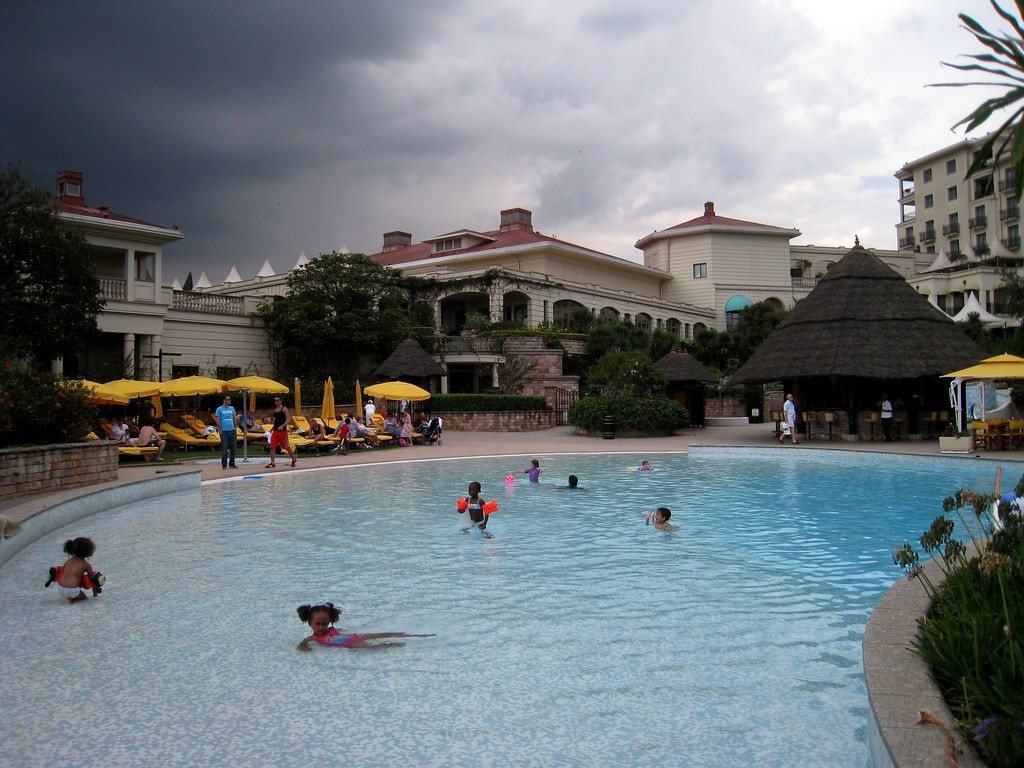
[223,399,231,401]
[274,399,280,402]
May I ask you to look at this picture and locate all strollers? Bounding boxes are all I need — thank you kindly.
[419,415,446,447]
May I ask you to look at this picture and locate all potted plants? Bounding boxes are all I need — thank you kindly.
[939,422,975,453]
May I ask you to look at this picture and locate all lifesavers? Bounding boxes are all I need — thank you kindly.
[42,565,63,588]
[483,500,498,515]
[627,466,640,471]
[457,499,468,510]
[82,571,107,597]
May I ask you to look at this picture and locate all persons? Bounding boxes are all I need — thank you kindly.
[510,459,544,483]
[335,400,383,454]
[265,399,297,467]
[295,602,438,652]
[557,475,586,490]
[644,506,676,533]
[387,400,437,445]
[303,418,324,441]
[457,480,497,540]
[215,395,239,470]
[108,415,167,462]
[637,460,654,472]
[778,393,801,446]
[881,394,894,442]
[56,537,99,605]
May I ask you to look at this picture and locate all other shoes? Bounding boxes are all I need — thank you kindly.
[229,464,238,469]
[793,441,798,444]
[778,438,784,444]
[291,458,297,467]
[265,463,275,468]
[222,465,226,470]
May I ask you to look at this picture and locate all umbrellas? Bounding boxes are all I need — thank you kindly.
[323,377,337,427]
[354,381,431,417]
[939,353,1024,433]
[295,378,302,416]
[171,245,351,291]
[53,374,289,424]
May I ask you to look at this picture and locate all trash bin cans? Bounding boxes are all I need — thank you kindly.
[749,403,764,423]
[603,415,615,439]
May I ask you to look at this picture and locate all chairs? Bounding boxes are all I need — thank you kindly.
[769,410,950,442]
[83,412,423,462]
[973,418,1024,450]
